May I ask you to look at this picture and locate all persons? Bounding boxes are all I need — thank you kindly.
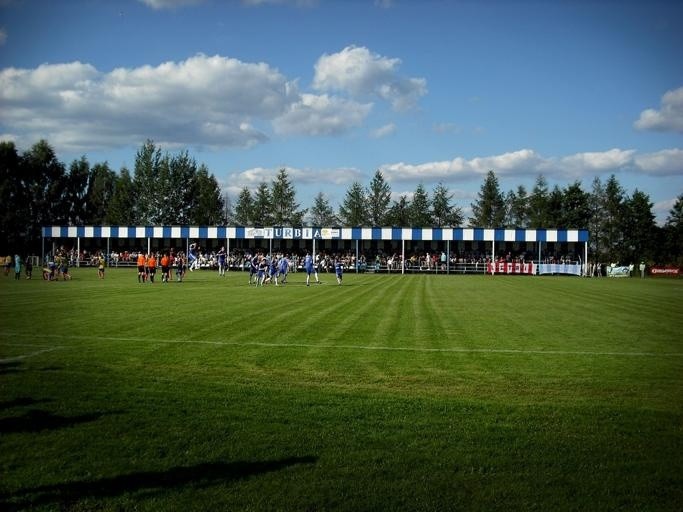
[248,248,357,286]
[137,248,187,283]
[629,261,634,278]
[639,259,645,279]
[43,246,148,283]
[188,246,253,277]
[461,249,582,270]
[404,249,458,274]
[588,261,603,278]
[1,254,32,280]
[358,248,403,274]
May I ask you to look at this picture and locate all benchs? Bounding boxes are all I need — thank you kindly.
[45,245,583,276]
